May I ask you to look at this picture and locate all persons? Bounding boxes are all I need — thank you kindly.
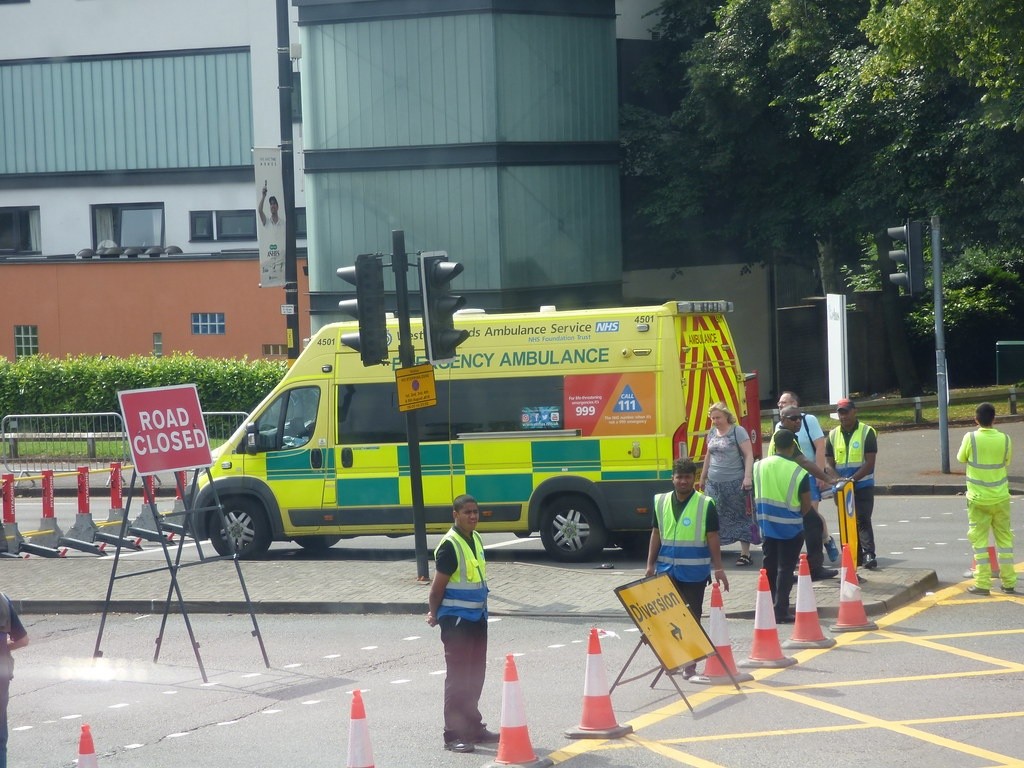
[0,591,29,768]
[752,429,812,624]
[644,457,730,680]
[956,402,1018,595]
[425,494,500,752]
[698,402,755,567]
[768,390,853,582]
[825,398,878,569]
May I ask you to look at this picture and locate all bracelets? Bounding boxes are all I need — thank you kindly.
[715,568,723,571]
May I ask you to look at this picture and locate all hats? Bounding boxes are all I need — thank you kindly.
[836,399,855,411]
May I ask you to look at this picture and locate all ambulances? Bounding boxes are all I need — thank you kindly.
[185,298,750,561]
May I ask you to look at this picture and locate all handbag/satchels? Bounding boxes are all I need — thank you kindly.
[743,480,754,491]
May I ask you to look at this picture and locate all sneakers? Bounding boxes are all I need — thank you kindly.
[472,728,500,744]
[967,586,990,596]
[444,739,476,752]
[824,536,839,562]
[1000,585,1015,594]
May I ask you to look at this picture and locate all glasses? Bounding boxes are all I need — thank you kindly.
[777,402,791,407]
[784,415,803,421]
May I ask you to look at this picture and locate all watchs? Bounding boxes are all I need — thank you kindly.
[849,477,855,482]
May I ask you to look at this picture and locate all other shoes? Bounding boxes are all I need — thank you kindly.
[811,568,838,582]
[793,575,798,583]
[776,614,796,624]
[666,668,678,675]
[683,670,696,679]
[857,552,877,569]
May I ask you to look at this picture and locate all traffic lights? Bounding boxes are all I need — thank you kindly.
[336,254,388,367]
[419,248,468,364]
[886,217,925,297]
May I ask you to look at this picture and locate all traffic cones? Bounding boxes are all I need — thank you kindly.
[345,690,375,768]
[780,553,837,649]
[78,724,99,768]
[734,568,798,669]
[479,652,554,768]
[963,524,1000,579]
[687,583,756,685]
[827,544,879,633]
[563,627,634,739]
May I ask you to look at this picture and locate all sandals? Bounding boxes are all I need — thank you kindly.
[736,555,753,566]
